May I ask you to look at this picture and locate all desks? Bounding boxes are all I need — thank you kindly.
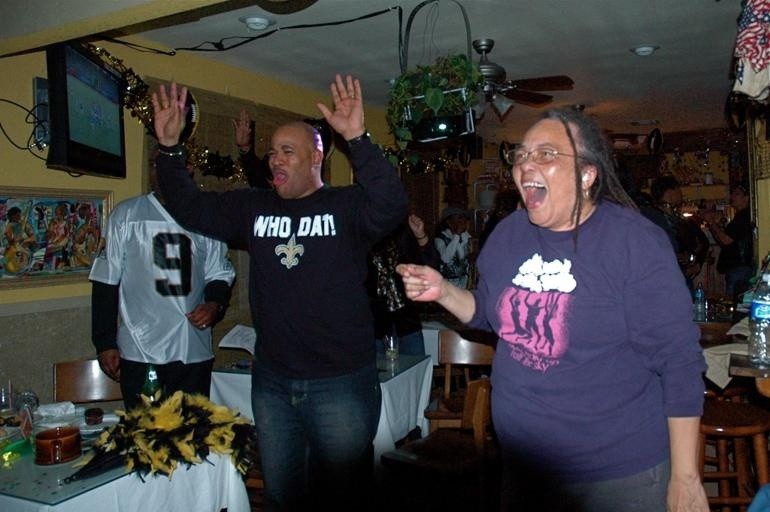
[698,345,770,380]
[209,352,433,510]
[0,401,252,511]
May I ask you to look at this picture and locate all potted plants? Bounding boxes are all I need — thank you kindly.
[383,55,482,172]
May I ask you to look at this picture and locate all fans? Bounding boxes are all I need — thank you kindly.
[470,39,574,119]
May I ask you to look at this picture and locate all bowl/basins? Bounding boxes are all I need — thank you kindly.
[35,426,82,466]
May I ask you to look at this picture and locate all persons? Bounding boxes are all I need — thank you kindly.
[151,74,410,512]
[4,203,74,272]
[638,175,710,304]
[367,215,441,357]
[702,184,756,298]
[88,142,237,417]
[396,109,710,511]
[431,206,473,291]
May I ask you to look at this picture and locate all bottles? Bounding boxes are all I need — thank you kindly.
[748,273,770,364]
[694,282,704,314]
[142,362,159,396]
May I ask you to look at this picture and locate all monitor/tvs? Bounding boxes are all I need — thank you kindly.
[404,87,476,143]
[45,44,128,180]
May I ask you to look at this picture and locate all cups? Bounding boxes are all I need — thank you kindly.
[384,336,400,360]
[84,407,104,426]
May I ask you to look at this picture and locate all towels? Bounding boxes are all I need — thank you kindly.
[36,400,75,419]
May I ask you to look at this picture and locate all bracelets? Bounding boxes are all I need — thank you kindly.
[414,233,427,241]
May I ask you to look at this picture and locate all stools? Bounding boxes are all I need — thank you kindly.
[688,397,770,512]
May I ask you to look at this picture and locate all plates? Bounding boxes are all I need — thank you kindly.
[70,414,125,435]
[1,419,23,442]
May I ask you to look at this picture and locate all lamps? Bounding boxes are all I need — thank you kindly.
[631,46,661,57]
[238,15,276,33]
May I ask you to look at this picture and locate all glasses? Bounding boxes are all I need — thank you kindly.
[501,146,583,166]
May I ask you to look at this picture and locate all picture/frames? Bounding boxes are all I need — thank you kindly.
[0,186,116,292]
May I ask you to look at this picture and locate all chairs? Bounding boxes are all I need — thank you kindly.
[439,330,498,402]
[380,378,499,509]
[51,357,129,412]
